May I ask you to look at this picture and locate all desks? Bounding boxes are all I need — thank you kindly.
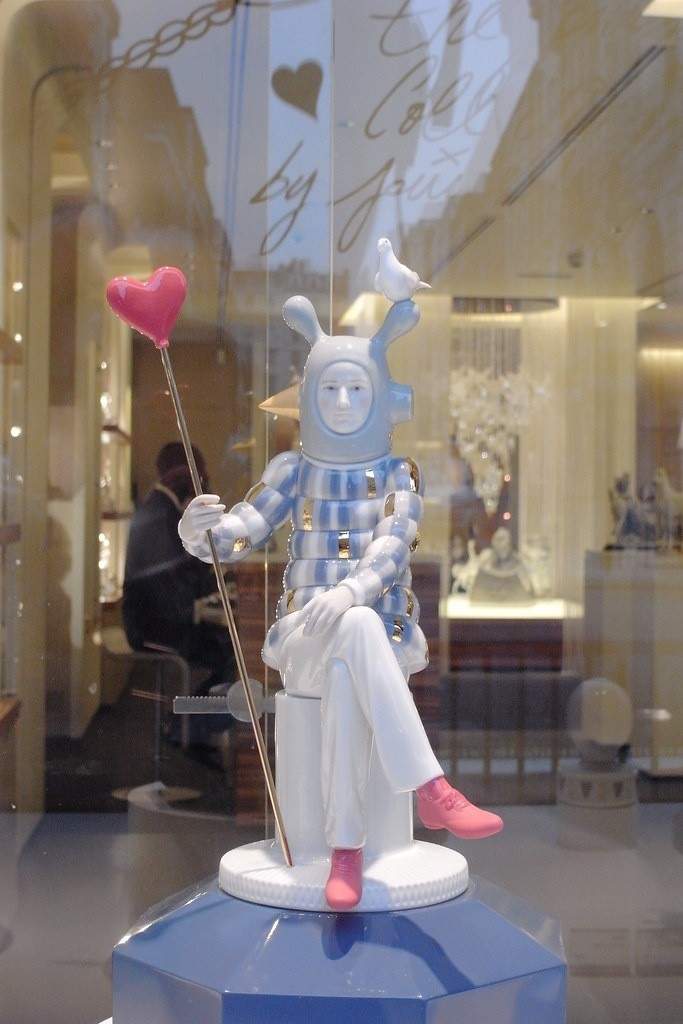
[236,552,446,827]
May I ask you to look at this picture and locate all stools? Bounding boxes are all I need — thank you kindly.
[92,623,211,805]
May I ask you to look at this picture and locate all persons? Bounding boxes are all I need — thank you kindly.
[121,438,238,805]
[176,294,503,909]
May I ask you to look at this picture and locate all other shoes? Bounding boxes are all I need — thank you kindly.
[167,731,182,746]
[189,742,223,767]
